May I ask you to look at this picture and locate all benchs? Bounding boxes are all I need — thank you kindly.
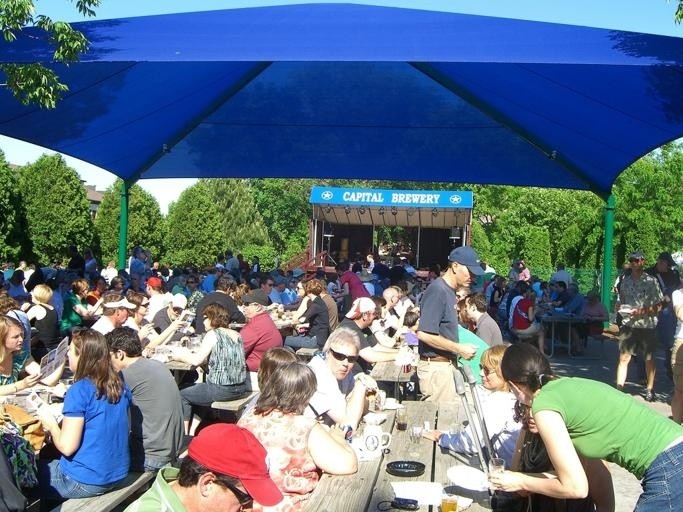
[210,391,259,411]
[43,469,153,512]
[297,347,318,356]
[585,334,610,346]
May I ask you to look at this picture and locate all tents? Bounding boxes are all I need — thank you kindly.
[0,1,683,332]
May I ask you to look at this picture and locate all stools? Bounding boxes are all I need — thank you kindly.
[517,334,535,343]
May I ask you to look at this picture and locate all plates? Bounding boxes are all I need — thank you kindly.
[385,461,425,475]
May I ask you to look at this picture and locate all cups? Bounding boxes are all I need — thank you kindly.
[489,458,505,486]
[191,336,202,352]
[145,346,156,359]
[182,337,192,349]
[441,487,457,512]
[397,406,408,428]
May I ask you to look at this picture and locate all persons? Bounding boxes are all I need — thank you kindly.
[101,261,119,286]
[482,275,500,300]
[671,287,683,425]
[550,263,573,286]
[515,259,531,283]
[303,328,382,435]
[195,276,247,338]
[0,294,33,367]
[466,292,504,346]
[416,245,480,401]
[550,284,559,302]
[129,247,148,291]
[127,292,186,354]
[124,423,283,512]
[103,327,186,475]
[87,276,105,307]
[107,277,125,297]
[235,364,358,512]
[283,280,330,353]
[570,290,608,355]
[335,262,369,305]
[528,276,550,303]
[66,245,86,279]
[506,281,527,319]
[60,278,105,339]
[21,285,59,363]
[317,281,338,335]
[3,260,64,281]
[199,253,332,295]
[543,279,571,307]
[5,270,25,298]
[241,346,305,421]
[508,288,533,330]
[640,253,682,385]
[359,238,439,290]
[672,268,683,291]
[84,249,98,276]
[335,298,405,379]
[239,289,283,393]
[456,287,476,333]
[494,399,615,512]
[116,269,132,290]
[508,262,523,286]
[554,284,586,316]
[289,281,313,322]
[22,329,133,499]
[498,283,512,327]
[0,315,41,396]
[153,292,197,343]
[513,289,550,358]
[178,302,249,436]
[488,343,683,512]
[90,294,153,352]
[613,252,663,403]
[368,284,422,345]
[490,278,505,313]
[146,277,173,323]
[419,345,525,471]
[146,259,204,296]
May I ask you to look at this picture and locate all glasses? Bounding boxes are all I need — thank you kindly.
[187,280,197,285]
[478,363,498,374]
[213,478,255,507]
[290,280,295,284]
[264,284,273,287]
[137,303,149,308]
[329,347,358,363]
[203,317,209,321]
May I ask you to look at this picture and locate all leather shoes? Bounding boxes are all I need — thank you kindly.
[646,389,655,400]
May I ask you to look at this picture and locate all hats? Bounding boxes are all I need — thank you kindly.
[187,423,284,507]
[145,277,162,287]
[102,297,136,310]
[658,252,676,265]
[629,252,645,260]
[345,297,377,319]
[242,289,268,306]
[171,294,187,310]
[448,246,487,275]
